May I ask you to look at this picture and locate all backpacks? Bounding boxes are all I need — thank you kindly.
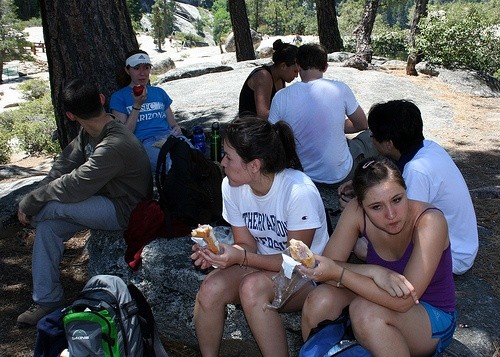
[299,304,373,357]
[62,274,169,357]
[155,134,231,237]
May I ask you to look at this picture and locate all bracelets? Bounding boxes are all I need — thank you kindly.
[132,105,141,110]
[337,267,348,286]
[241,249,248,269]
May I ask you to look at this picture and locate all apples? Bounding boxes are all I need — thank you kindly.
[133,84,144,96]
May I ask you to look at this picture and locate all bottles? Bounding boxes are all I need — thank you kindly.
[192,126,206,154]
[211,122,221,162]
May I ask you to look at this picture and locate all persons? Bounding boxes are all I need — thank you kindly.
[267,43,381,188]
[336,98,478,275]
[109,50,199,171]
[191,116,329,357]
[12,77,151,329]
[237,38,300,120]
[296,157,458,357]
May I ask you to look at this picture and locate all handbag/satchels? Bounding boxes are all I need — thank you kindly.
[33,306,69,357]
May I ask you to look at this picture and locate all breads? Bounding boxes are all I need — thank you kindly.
[190,224,219,254]
[289,238,314,268]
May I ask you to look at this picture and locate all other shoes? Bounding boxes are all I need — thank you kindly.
[17,303,47,325]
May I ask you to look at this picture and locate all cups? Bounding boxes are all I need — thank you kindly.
[338,186,355,211]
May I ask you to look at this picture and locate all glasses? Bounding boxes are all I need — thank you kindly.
[368,129,378,138]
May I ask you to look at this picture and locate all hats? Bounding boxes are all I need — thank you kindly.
[126,53,153,67]
